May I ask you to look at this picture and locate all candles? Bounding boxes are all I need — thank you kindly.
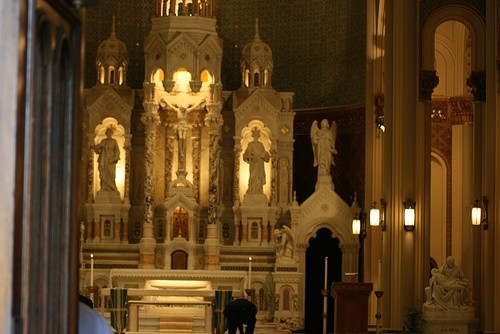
[90,254,94,286]
[249,256,252,288]
[378,260,381,291]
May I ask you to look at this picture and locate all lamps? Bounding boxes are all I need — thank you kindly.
[471,196,489,230]
[352,212,366,237]
[325,256,328,290]
[403,199,416,231]
[369,199,387,232]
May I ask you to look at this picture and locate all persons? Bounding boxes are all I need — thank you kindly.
[78,297,111,334]
[92,127,120,191]
[223,299,257,334]
[314,118,338,176]
[243,129,270,195]
[428,257,476,310]
[160,97,206,156]
